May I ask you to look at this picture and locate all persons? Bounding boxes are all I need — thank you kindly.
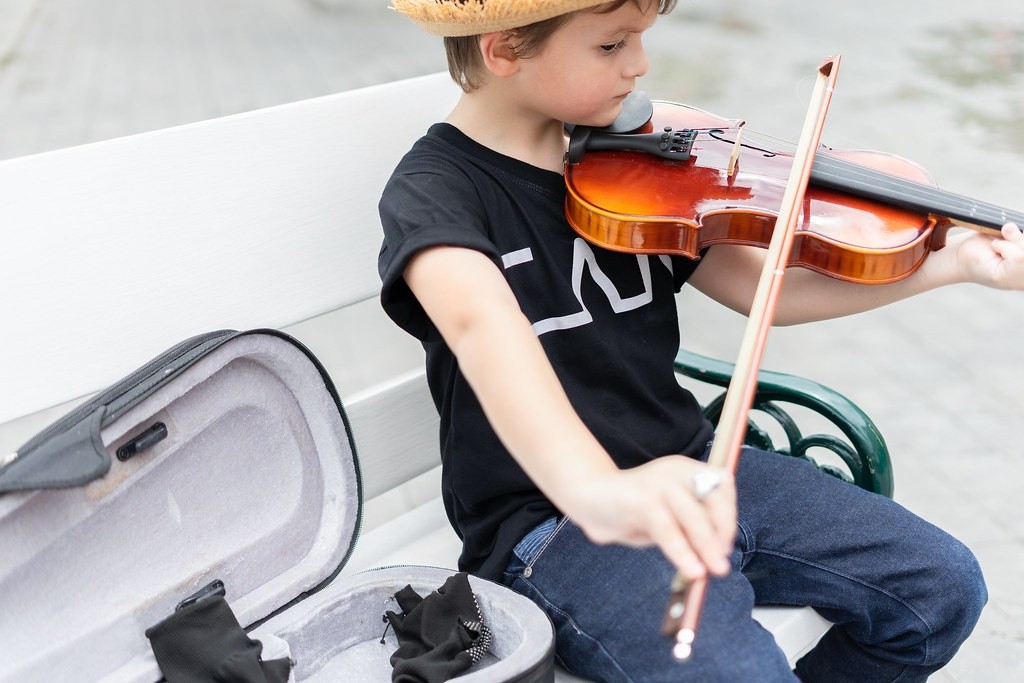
[378,0,1023,683]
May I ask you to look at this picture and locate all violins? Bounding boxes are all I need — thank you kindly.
[565,99,1024,286]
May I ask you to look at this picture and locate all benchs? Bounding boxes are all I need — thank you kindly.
[0,72,892,683]
